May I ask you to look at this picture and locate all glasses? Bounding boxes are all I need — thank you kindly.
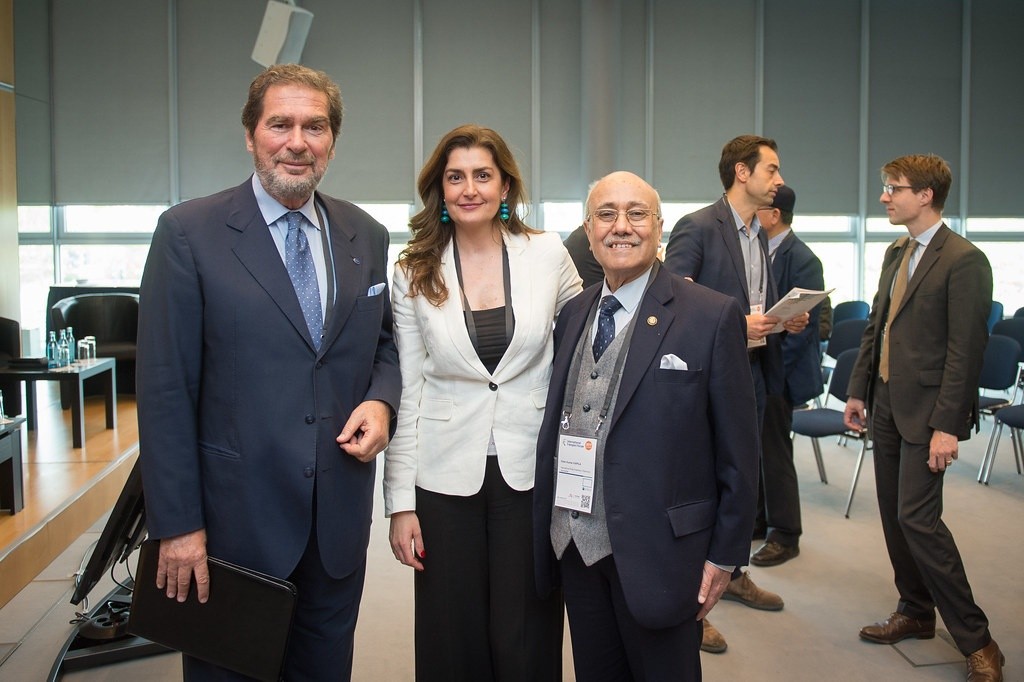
[882,184,912,196]
[587,207,659,227]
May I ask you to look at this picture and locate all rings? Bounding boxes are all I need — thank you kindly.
[946,458,953,464]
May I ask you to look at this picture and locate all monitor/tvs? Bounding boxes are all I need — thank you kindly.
[70,454,150,607]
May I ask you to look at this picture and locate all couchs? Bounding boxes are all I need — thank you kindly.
[50,291,139,410]
[0,317,20,418]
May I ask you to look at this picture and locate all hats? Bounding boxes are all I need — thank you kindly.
[769,185,795,211]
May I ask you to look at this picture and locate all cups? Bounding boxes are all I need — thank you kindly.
[78,336,96,368]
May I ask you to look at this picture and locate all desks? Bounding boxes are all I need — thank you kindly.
[0,415,27,515]
[0,357,117,448]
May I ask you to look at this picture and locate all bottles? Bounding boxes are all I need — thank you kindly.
[47,327,75,369]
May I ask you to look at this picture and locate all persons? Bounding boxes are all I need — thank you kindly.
[532,169,762,682]
[133,62,404,682]
[553,215,665,326]
[749,181,825,569]
[382,122,586,682]
[664,133,811,654]
[813,285,835,357]
[842,151,1008,682]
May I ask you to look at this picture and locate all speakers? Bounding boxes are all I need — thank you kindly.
[251,0,314,68]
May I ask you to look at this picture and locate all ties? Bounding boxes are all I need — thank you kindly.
[879,239,920,383]
[285,211,323,352]
[593,296,623,364]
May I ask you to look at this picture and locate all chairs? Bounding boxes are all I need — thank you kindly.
[791,301,1024,518]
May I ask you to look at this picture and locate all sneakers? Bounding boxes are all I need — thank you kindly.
[701,617,728,653]
[719,571,784,611]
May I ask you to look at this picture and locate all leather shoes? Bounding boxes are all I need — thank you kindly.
[966,639,1005,682]
[750,542,800,566]
[859,611,935,644]
